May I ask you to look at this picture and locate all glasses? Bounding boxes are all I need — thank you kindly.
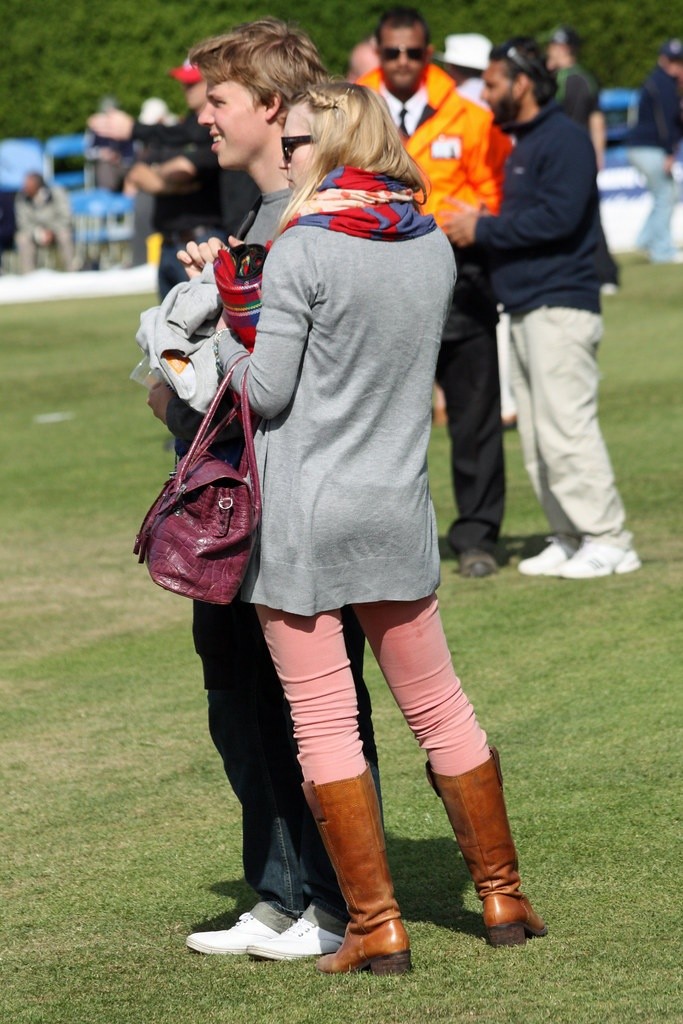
[282,136,314,162]
[506,43,536,77]
[385,48,423,60]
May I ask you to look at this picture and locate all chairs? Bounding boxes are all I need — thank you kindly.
[65,188,135,273]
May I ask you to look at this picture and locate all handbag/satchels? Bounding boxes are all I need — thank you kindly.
[134,355,260,608]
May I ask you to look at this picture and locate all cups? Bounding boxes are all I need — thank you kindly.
[129,354,172,391]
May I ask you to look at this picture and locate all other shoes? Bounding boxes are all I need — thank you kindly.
[459,545,499,577]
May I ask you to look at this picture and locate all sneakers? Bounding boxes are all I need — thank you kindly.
[560,541,641,580]
[249,916,345,961]
[518,535,573,577]
[187,914,269,956]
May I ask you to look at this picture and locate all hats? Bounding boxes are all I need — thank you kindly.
[171,56,203,84]
[554,27,580,54]
[444,33,491,70]
[662,38,683,63]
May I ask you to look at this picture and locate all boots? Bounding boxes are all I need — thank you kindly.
[299,765,412,976]
[427,745,548,947]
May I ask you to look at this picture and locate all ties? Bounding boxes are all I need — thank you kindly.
[399,108,410,139]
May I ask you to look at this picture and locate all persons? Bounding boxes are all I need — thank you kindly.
[344,5,683,579]
[213,81,547,975]
[147,19,368,962]
[14,95,188,272]
